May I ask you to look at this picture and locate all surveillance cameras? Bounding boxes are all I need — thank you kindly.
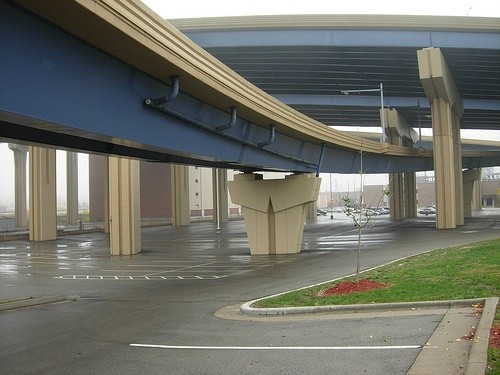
[425,114,432,121]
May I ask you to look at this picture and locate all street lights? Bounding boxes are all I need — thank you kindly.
[340,83,386,143]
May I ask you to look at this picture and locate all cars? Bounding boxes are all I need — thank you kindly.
[316,204,437,221]
